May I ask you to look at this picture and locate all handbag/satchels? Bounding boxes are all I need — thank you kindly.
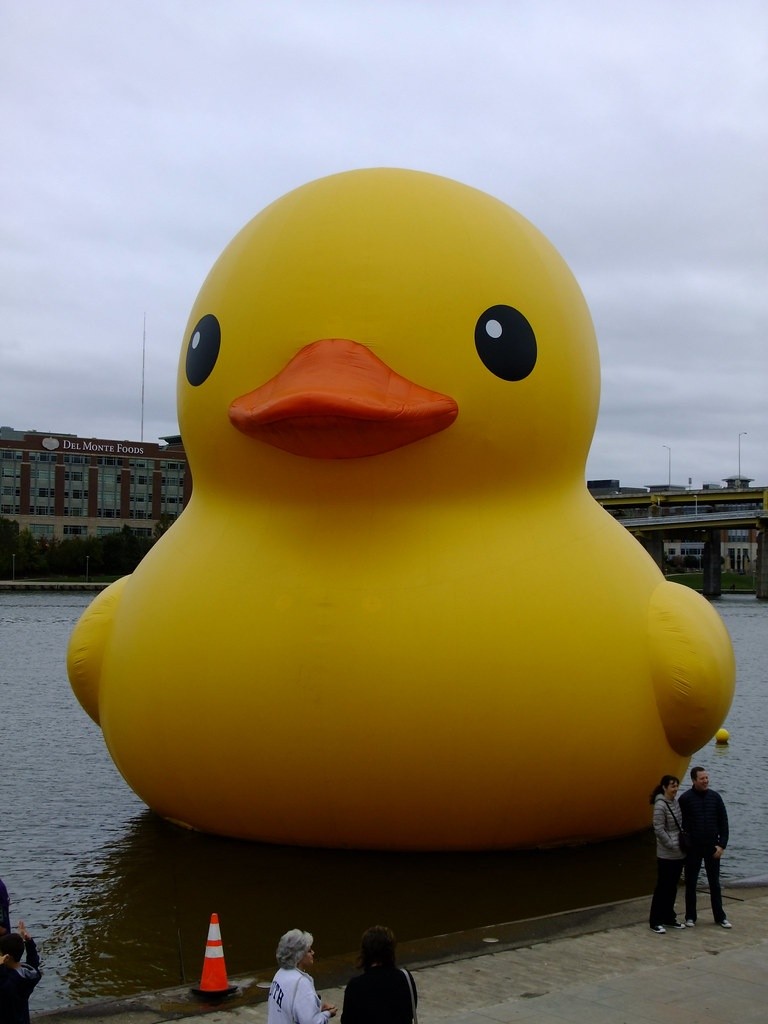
[678,830,718,859]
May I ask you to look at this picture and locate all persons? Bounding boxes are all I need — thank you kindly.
[267,929,337,1024]
[0,879,42,1024]
[650,775,686,933]
[341,927,417,1024]
[679,767,732,928]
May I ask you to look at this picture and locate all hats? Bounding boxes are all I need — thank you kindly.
[276,928,314,970]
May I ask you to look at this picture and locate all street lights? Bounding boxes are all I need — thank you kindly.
[662,445,671,485]
[12,554,16,580]
[739,432,747,477]
[693,495,697,514]
[86,556,90,582]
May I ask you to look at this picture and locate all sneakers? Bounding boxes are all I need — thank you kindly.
[685,919,696,926]
[649,923,667,933]
[715,918,732,928]
[667,921,685,929]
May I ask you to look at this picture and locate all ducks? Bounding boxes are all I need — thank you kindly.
[64,165,740,869]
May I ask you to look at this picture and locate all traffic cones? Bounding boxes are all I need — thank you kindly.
[189,913,238,1000]
